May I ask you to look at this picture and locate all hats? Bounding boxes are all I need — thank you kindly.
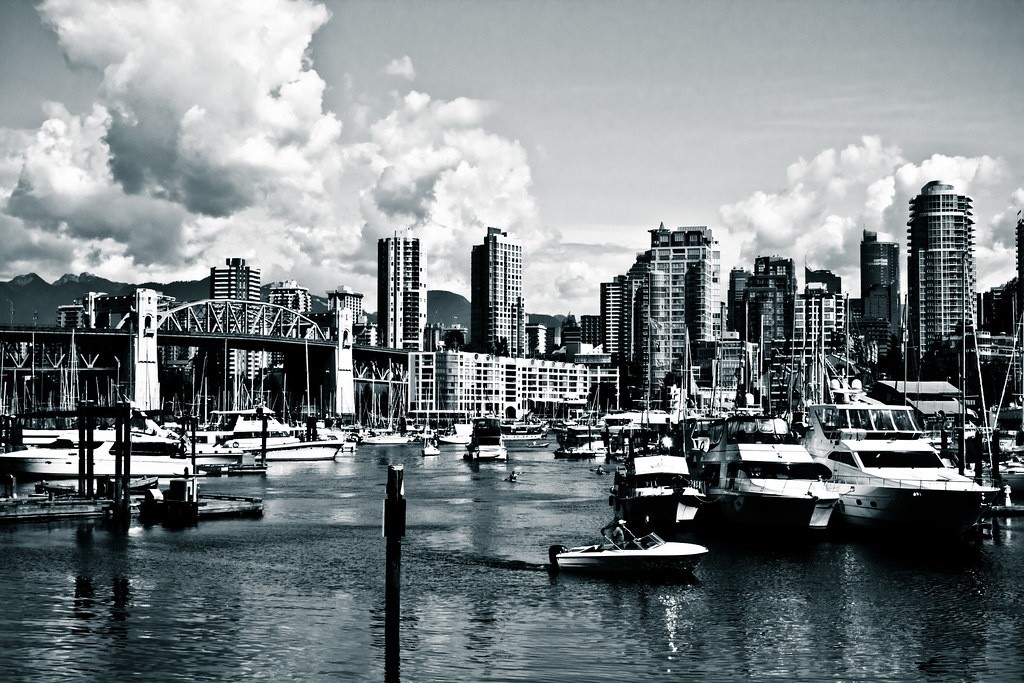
[618,520,626,523]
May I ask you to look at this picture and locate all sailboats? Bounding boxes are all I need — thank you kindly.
[932,259,1023,492]
[343,381,550,462]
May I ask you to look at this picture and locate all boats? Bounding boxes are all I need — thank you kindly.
[185,406,344,461]
[33,475,159,494]
[800,404,1002,532]
[675,415,855,532]
[0,428,208,482]
[610,431,708,530]
[551,364,673,459]
[549,518,710,574]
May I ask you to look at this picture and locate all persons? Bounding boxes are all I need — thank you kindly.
[510,470,517,481]
[660,430,675,452]
[600,516,629,550]
[598,465,604,474]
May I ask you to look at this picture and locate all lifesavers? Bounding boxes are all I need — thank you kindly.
[936,410,946,423]
